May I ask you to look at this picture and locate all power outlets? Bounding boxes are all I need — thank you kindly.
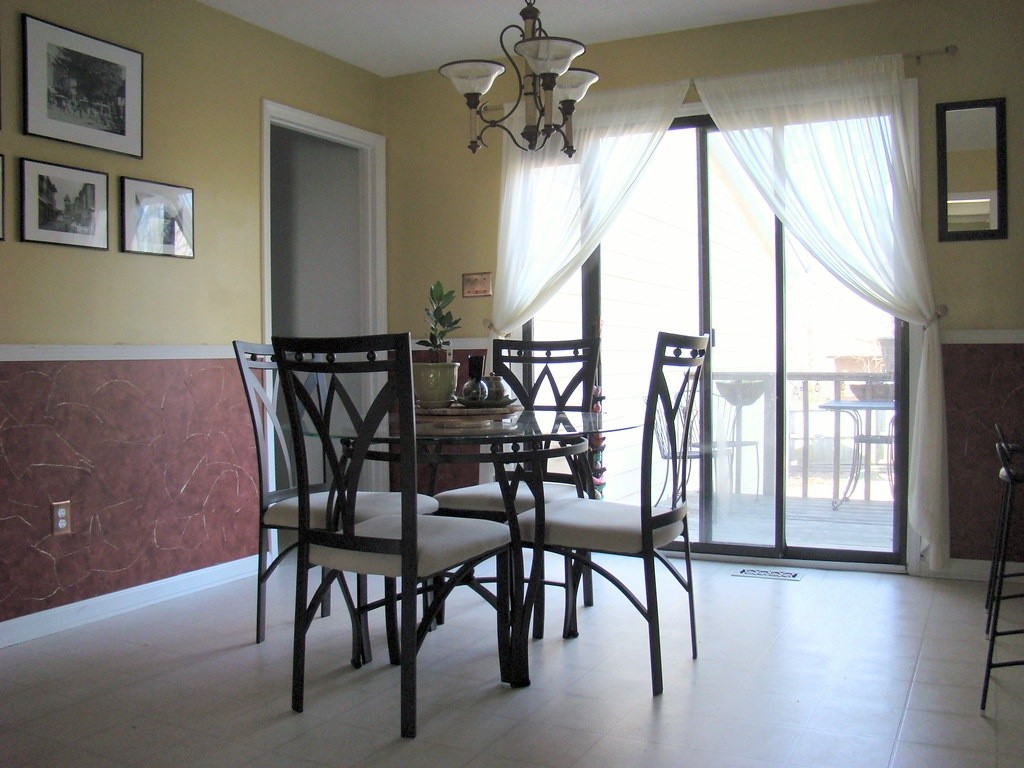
[52,500,71,535]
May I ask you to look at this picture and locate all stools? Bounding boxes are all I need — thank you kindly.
[979,422,1024,716]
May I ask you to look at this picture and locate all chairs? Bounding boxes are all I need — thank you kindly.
[504,332,708,695]
[433,337,601,639]
[643,390,760,522]
[233,340,439,664]
[271,331,512,738]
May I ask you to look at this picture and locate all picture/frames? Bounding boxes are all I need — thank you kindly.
[19,157,109,251]
[21,13,145,159]
[121,176,194,259]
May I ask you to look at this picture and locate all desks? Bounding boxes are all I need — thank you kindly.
[282,411,645,689]
[820,401,896,510]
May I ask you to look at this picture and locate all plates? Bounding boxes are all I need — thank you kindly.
[457,394,518,408]
[416,400,455,409]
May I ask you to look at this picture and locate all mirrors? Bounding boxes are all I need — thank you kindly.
[935,97,1008,242]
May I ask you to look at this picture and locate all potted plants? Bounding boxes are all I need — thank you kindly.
[410,280,462,409]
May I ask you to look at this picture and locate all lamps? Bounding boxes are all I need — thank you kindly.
[439,0,600,158]
